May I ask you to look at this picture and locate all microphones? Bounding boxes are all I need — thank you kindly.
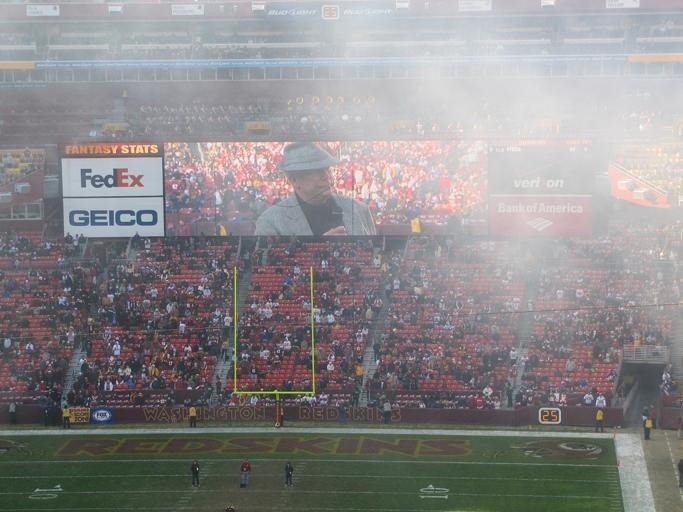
[332,207,343,229]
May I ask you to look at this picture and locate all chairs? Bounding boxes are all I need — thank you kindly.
[0,229,683,408]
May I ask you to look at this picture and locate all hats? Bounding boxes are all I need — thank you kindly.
[276,142,339,170]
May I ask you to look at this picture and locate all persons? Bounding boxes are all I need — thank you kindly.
[238,457,252,488]
[1,138,682,440]
[190,456,200,487]
[284,462,293,487]
